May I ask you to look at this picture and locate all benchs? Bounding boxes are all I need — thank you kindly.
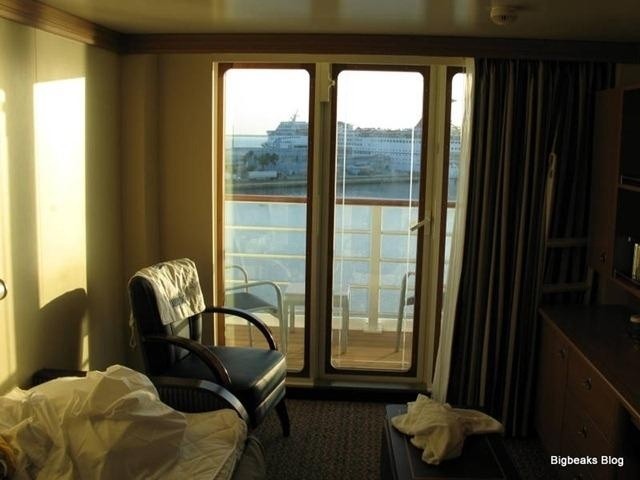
[0,369,268,480]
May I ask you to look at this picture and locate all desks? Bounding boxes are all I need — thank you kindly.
[382,403,521,480]
[282,282,350,354]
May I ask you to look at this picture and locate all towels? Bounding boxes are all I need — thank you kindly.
[391,394,505,465]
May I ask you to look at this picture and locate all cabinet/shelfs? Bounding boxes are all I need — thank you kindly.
[535,86,640,480]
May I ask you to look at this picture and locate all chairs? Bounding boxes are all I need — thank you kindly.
[396,271,415,354]
[130,257,291,438]
[224,264,285,357]
